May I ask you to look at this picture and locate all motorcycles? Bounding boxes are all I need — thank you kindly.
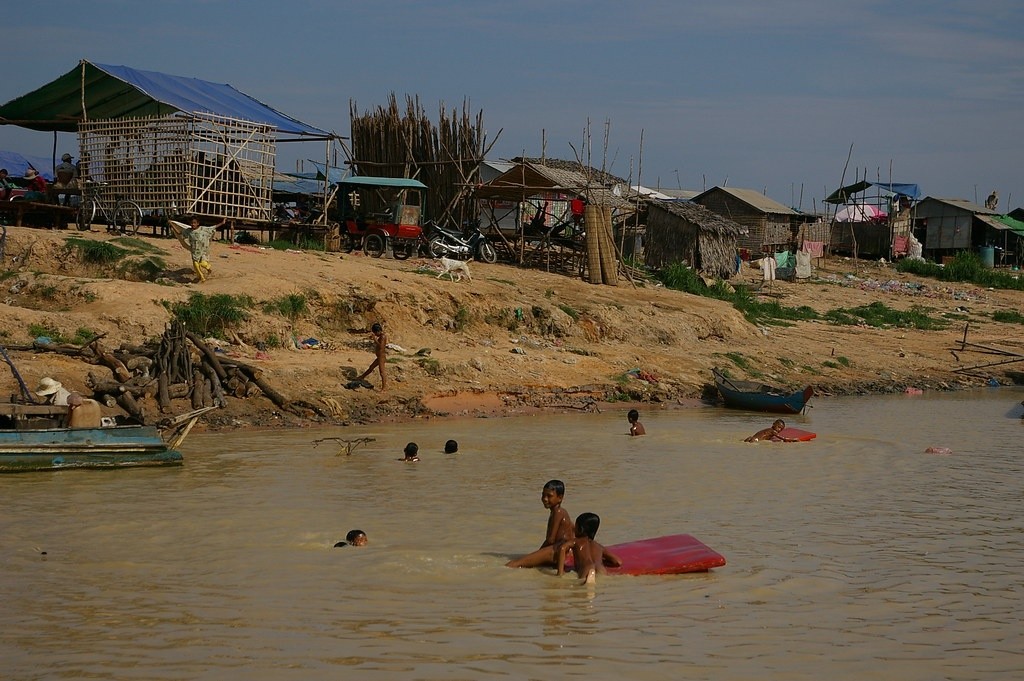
[428,219,498,264]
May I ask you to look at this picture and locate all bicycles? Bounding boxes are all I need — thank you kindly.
[75,175,143,236]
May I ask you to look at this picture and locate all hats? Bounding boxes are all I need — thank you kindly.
[34,376,62,396]
[22,170,36,179]
[62,153,74,160]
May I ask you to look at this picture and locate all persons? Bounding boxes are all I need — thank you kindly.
[346,530,368,546]
[398,442,420,462]
[505,479,575,568]
[745,419,799,443]
[105,145,118,180]
[56,153,79,177]
[36,377,75,409]
[23,169,46,193]
[628,410,646,436]
[168,216,227,282]
[358,323,386,394]
[556,513,622,584]
[445,440,458,454]
[75,153,90,180]
[0,168,8,181]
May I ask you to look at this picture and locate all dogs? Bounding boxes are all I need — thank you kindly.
[435,256,473,286]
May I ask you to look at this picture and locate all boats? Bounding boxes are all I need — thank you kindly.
[710,367,814,414]
